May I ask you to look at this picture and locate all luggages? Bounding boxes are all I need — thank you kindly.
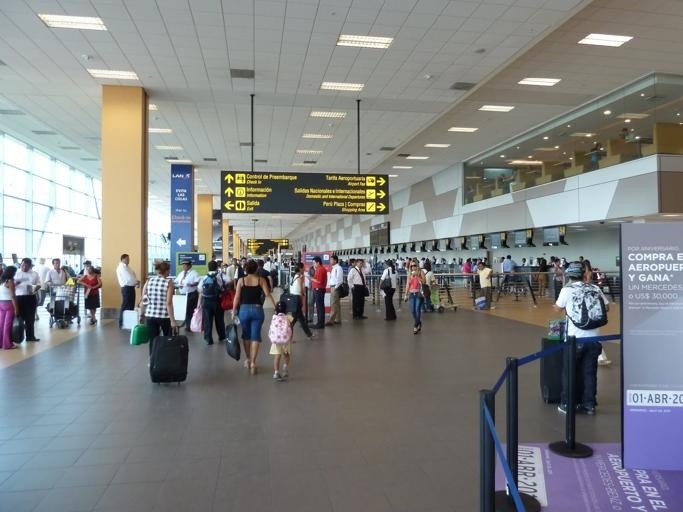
[149,326,188,385]
[539,337,561,405]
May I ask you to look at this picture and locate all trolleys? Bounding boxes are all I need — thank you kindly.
[428,280,458,313]
[46,282,82,327]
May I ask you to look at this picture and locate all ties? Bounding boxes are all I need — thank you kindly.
[179,271,186,289]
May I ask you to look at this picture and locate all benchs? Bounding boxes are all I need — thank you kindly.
[469,117,683,203]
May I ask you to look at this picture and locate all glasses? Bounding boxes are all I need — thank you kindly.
[410,265,416,267]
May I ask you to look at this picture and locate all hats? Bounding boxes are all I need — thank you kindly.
[563,262,583,278]
[82,260,90,265]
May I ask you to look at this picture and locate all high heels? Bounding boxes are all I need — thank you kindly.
[90,320,96,325]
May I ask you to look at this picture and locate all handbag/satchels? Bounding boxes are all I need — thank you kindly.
[379,269,392,289]
[474,296,487,310]
[55,285,76,302]
[547,319,565,338]
[225,324,241,360]
[338,283,349,298]
[363,287,369,296]
[418,283,431,298]
[189,307,203,333]
[11,314,24,343]
[279,294,300,313]
[130,320,150,345]
[219,288,236,310]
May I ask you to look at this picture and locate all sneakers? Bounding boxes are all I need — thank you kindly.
[557,404,567,414]
[575,403,595,415]
[272,374,282,379]
[282,367,288,378]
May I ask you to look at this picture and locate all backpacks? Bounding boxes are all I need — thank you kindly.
[202,272,221,300]
[564,282,610,330]
[268,313,292,345]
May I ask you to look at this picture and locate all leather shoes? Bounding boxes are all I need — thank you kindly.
[413,327,417,334]
[417,322,420,331]
[353,315,368,320]
[26,337,39,341]
[313,320,342,328]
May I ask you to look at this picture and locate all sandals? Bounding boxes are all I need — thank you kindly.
[250,367,256,375]
[243,360,250,370]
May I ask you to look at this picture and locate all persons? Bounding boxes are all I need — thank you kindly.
[555,262,610,415]
[173,255,612,379]
[140,262,176,367]
[116,254,141,330]
[0,258,101,350]
